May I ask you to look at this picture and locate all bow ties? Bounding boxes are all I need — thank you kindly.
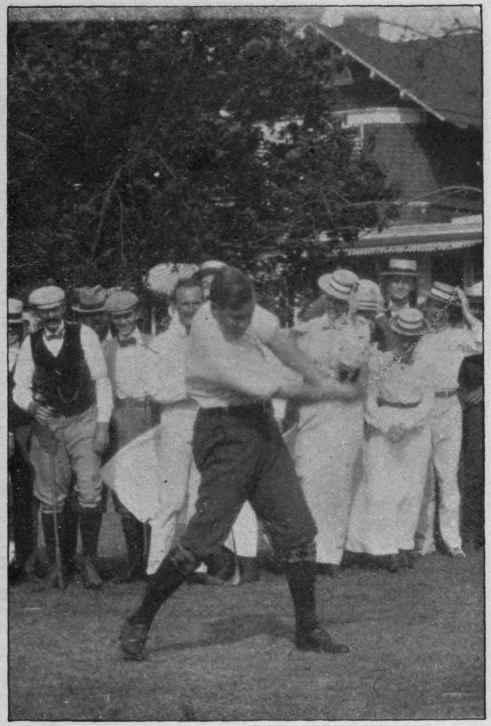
[47,335,61,341]
[119,337,136,346]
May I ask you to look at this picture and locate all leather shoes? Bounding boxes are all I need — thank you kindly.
[9,553,160,589]
[122,616,152,660]
[224,569,260,585]
[450,544,466,560]
[297,628,350,653]
[317,541,436,571]
[186,573,224,587]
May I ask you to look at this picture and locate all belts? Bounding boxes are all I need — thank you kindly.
[435,392,457,398]
[379,396,421,408]
[197,400,272,416]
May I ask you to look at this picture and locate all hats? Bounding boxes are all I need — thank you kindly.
[104,290,140,317]
[72,283,107,312]
[7,297,25,324]
[28,286,65,309]
[379,257,420,277]
[192,259,227,279]
[318,270,357,304]
[351,280,383,314]
[387,308,431,337]
[422,280,483,309]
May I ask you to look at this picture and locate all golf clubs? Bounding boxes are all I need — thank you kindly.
[33,392,66,588]
[230,528,241,586]
